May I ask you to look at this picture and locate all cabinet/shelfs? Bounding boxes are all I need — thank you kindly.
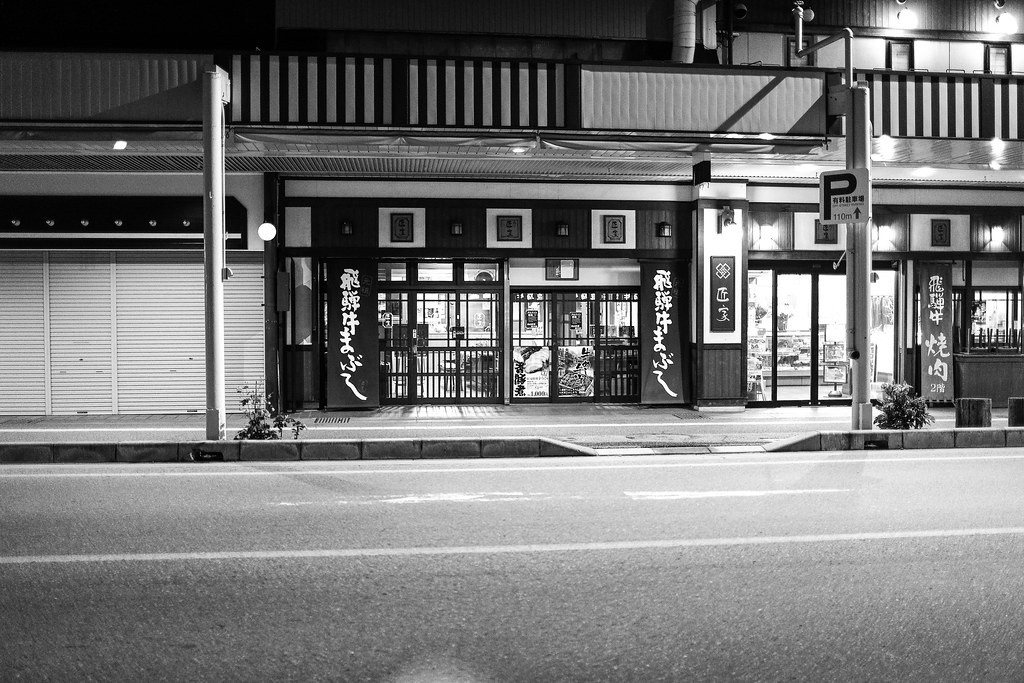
[767,324,843,387]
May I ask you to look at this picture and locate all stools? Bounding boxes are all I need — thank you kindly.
[957,327,1024,355]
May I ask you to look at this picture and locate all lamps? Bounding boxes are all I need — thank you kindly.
[341,218,352,236]
[451,221,464,236]
[658,222,672,236]
[557,222,569,236]
[257,217,277,241]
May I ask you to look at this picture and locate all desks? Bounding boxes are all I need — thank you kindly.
[441,362,464,391]
